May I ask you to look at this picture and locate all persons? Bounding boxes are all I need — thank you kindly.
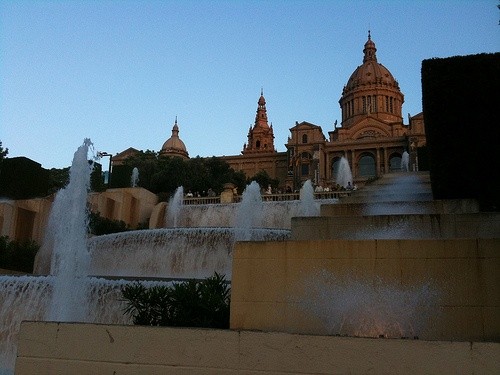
[186,179,360,206]
